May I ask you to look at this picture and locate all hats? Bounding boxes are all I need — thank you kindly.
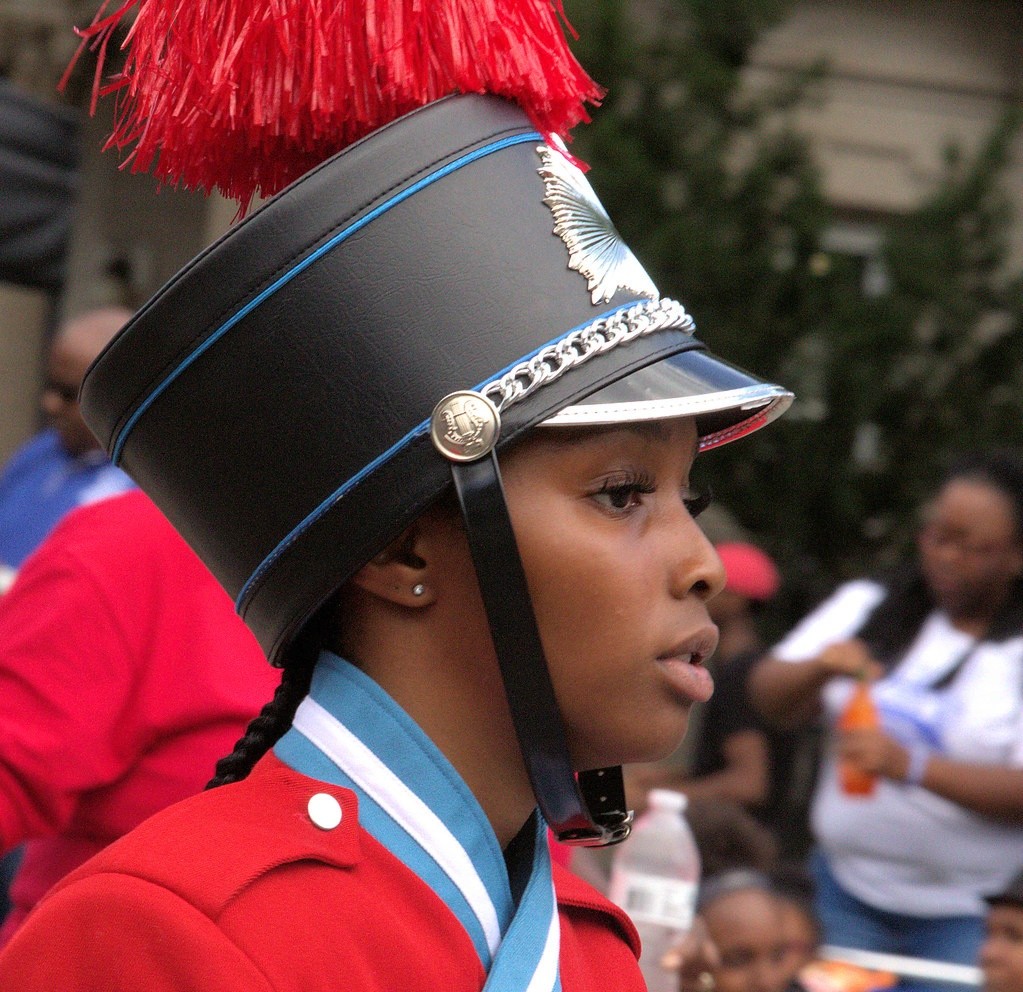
[79,3,797,667]
[710,540,780,598]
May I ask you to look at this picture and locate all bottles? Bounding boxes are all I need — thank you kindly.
[606,785,702,992]
[837,667,880,799]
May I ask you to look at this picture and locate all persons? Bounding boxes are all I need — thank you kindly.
[1,287,1022,984]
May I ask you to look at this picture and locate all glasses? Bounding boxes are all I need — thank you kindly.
[39,361,82,403]
[920,507,1011,555]
